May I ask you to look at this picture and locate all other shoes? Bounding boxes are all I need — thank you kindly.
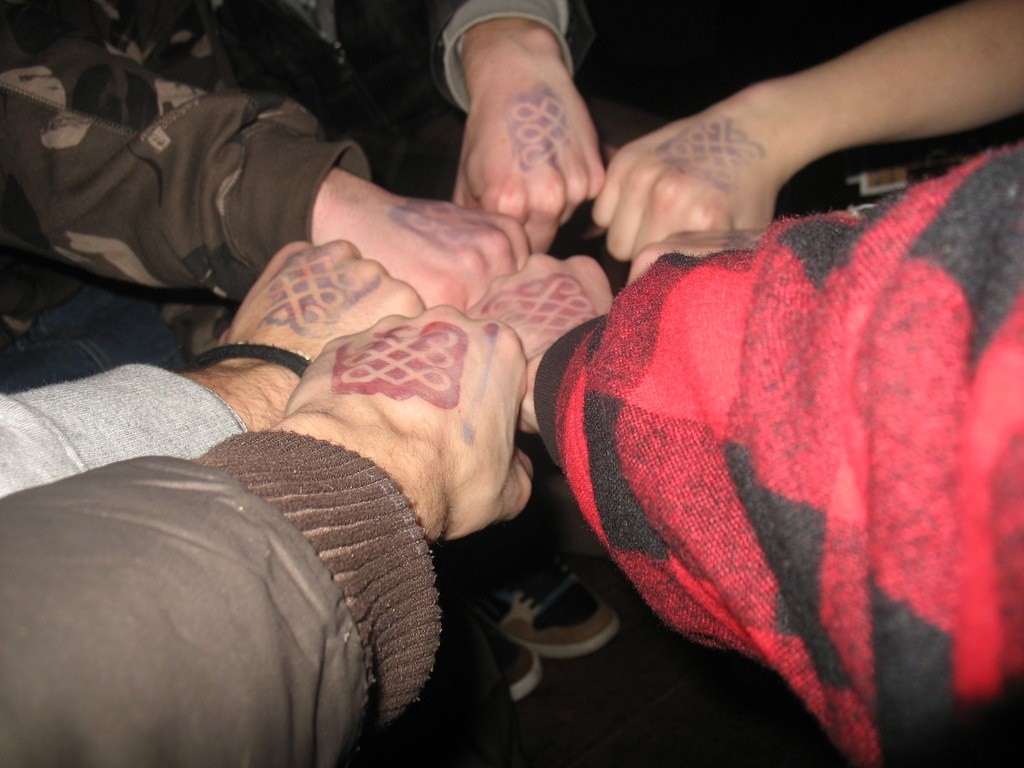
[472,627,543,700]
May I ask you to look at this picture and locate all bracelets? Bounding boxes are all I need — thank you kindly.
[192,341,313,377]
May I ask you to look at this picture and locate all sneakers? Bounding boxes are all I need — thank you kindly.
[477,556,619,656]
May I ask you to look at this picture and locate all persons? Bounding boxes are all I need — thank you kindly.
[1,0,1023,767]
